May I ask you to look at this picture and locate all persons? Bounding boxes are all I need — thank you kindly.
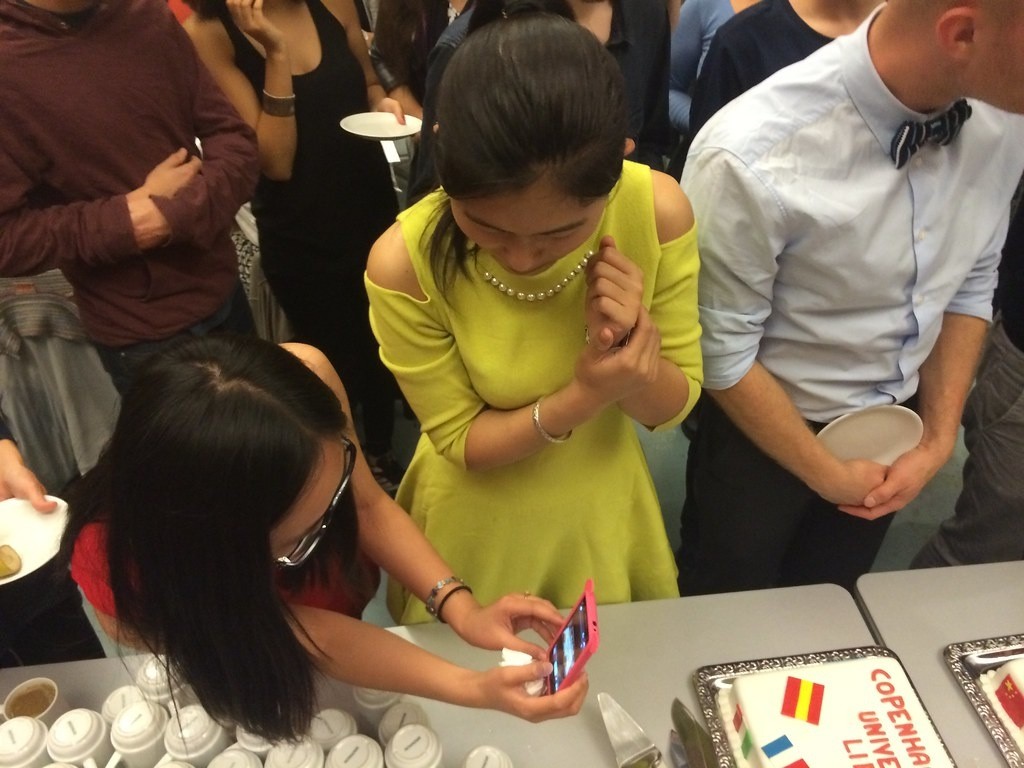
[0,0,887,661]
[55,331,589,724]
[910,175,1023,569]
[363,0,705,626]
[681,0,1024,596]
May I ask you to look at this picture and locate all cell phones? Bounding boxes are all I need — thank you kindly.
[541,579,598,696]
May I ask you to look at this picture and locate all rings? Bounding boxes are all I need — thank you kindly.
[524,590,531,599]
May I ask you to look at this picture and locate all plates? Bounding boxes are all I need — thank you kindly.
[692,646,959,768]
[816,405,924,468]
[943,634,1024,768]
[340,112,422,142]
[0,495,69,586]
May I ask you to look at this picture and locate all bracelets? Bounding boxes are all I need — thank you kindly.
[262,89,296,117]
[583,325,630,351]
[437,586,472,623]
[425,577,466,618]
[533,393,573,442]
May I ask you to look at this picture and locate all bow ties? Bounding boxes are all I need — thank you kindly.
[891,97,973,170]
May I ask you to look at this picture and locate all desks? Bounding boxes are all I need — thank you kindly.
[0,581,911,767]
[854,560,1024,768]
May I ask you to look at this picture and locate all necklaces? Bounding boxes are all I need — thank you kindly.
[473,249,594,302]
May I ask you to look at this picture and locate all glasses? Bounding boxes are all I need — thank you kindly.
[274,435,357,574]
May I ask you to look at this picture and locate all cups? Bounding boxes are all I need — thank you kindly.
[0,651,445,768]
[462,744,513,768]
